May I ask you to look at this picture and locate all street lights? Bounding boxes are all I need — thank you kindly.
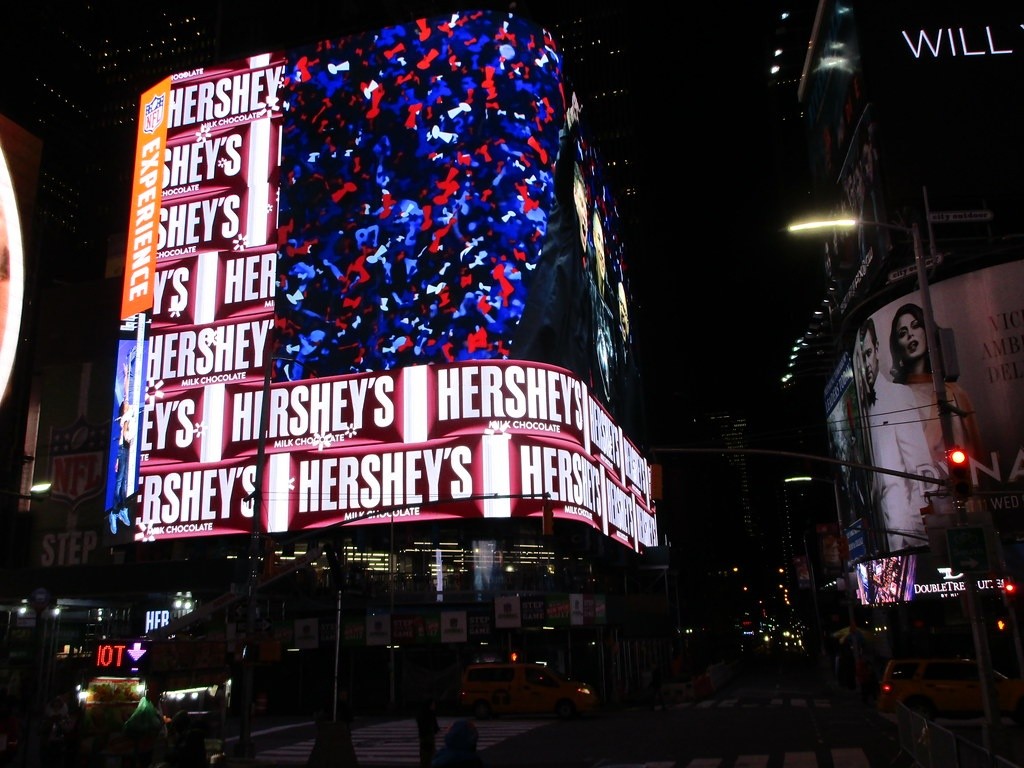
[784,208,1006,760]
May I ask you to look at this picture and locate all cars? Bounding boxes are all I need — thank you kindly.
[878,659,1023,725]
[460,664,598,721]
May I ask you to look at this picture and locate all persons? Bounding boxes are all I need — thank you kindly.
[852,304,984,552]
[416,697,439,768]
[429,718,485,768]
[0,667,212,768]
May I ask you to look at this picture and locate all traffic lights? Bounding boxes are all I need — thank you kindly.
[645,464,663,501]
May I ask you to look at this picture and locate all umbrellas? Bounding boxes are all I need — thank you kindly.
[833,627,874,646]
